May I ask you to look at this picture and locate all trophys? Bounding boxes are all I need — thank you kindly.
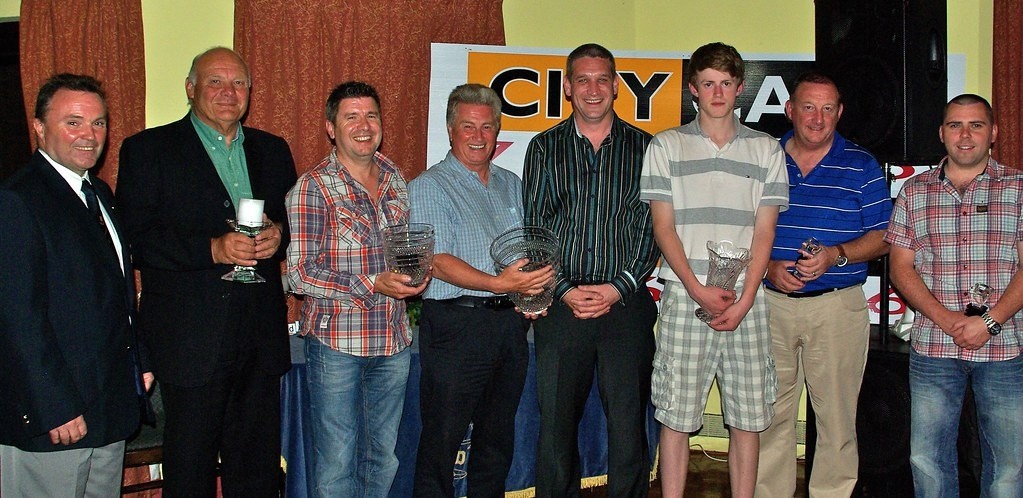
[791,236,823,284]
[963,282,995,316]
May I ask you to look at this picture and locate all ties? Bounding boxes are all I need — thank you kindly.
[80,179,123,279]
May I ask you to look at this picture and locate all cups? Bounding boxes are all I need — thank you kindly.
[382,224,435,287]
[490,226,560,315]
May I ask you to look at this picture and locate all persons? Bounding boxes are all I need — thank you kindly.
[116,48,299,498]
[284,79,433,498]
[640,42,790,498]
[408,83,555,498]
[754,75,894,498]
[521,44,663,498]
[883,94,1023,498]
[0,69,154,498]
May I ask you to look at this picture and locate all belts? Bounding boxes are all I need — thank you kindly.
[765,282,855,298]
[452,296,515,309]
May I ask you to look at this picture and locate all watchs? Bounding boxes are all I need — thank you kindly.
[980,311,1001,336]
[834,243,848,268]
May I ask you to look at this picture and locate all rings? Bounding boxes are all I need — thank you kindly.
[812,272,816,276]
[542,283,551,292]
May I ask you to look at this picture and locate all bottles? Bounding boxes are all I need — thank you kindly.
[695,240,753,325]
